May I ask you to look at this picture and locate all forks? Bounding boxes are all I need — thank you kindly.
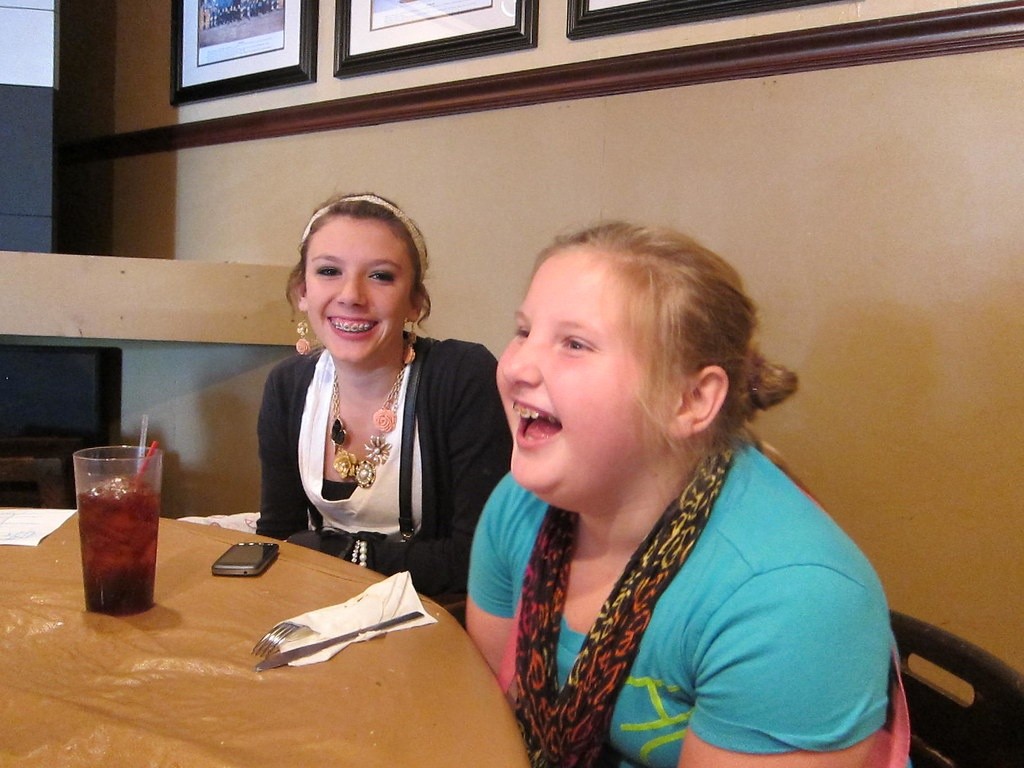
[251,619,320,659]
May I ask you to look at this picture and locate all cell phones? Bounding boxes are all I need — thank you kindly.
[211,542,279,575]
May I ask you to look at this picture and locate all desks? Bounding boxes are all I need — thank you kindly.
[0,507,530,768]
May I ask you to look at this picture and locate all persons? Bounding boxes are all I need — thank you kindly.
[464,225,911,767]
[254,194,513,630]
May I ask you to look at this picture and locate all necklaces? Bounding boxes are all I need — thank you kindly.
[330,364,407,489]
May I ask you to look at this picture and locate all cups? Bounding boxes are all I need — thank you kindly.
[73,445,164,617]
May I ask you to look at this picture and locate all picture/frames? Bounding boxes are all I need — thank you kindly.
[334,0,539,80]
[170,0,320,105]
[565,0,866,40]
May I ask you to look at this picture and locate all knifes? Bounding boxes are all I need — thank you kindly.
[253,611,425,670]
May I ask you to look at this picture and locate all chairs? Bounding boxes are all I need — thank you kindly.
[0,457,69,509]
[882,610,1024,768]
